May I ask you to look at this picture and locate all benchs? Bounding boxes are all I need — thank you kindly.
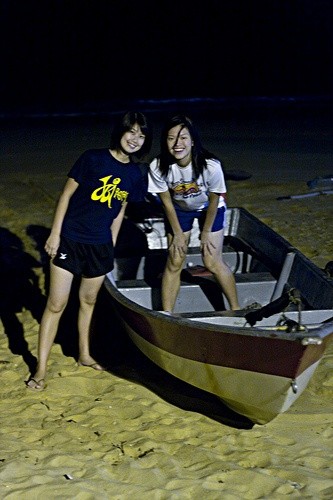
[114,271,277,314]
[115,242,256,279]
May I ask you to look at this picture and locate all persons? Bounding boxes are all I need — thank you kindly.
[147,115,242,315]
[25,111,153,392]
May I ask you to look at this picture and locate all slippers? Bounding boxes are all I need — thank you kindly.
[25,378,45,391]
[77,359,108,371]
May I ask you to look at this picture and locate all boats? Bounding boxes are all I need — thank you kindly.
[100,203,331,424]
